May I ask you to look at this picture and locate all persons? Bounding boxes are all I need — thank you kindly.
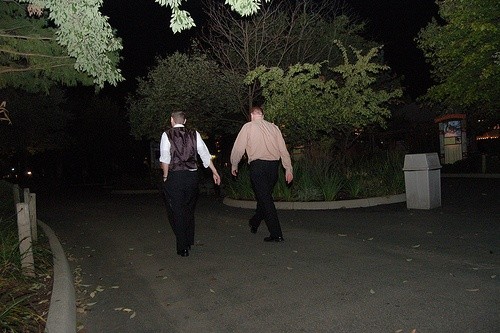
[159,108,221,258]
[229,106,294,242]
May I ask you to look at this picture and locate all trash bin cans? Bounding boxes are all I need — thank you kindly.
[402,152,443,210]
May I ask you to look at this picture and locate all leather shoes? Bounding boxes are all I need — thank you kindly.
[249,219,257,233]
[176,245,192,257]
[264,235,284,242]
